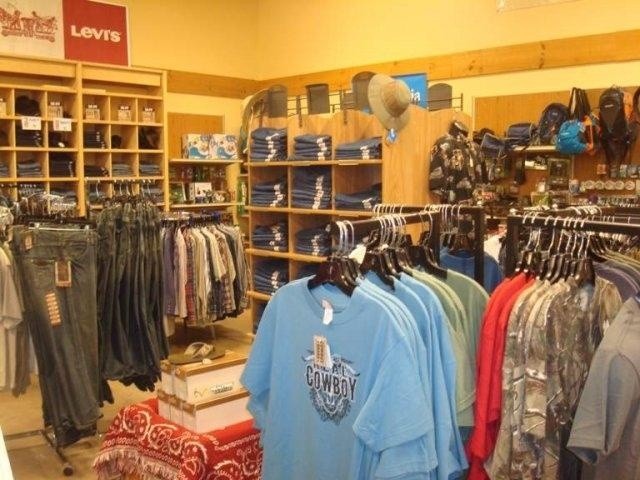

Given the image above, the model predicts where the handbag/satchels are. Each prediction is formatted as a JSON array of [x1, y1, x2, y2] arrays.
[[473, 89, 636, 161]]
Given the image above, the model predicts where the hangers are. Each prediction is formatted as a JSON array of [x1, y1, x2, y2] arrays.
[[0, 177, 162, 230], [359, 203, 474, 296], [308, 220, 364, 297], [490, 195, 637, 297]]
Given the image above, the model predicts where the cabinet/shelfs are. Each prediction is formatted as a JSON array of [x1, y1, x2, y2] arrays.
[[244, 103, 430, 346], [169, 157, 244, 211], [0, 54, 168, 219]]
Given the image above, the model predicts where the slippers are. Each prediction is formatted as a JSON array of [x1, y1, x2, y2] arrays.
[[168, 341, 224, 366]]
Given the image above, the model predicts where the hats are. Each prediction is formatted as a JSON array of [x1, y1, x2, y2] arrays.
[[367, 73, 410, 132]]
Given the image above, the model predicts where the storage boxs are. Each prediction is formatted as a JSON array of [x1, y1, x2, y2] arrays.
[[156, 348, 252, 432]]
